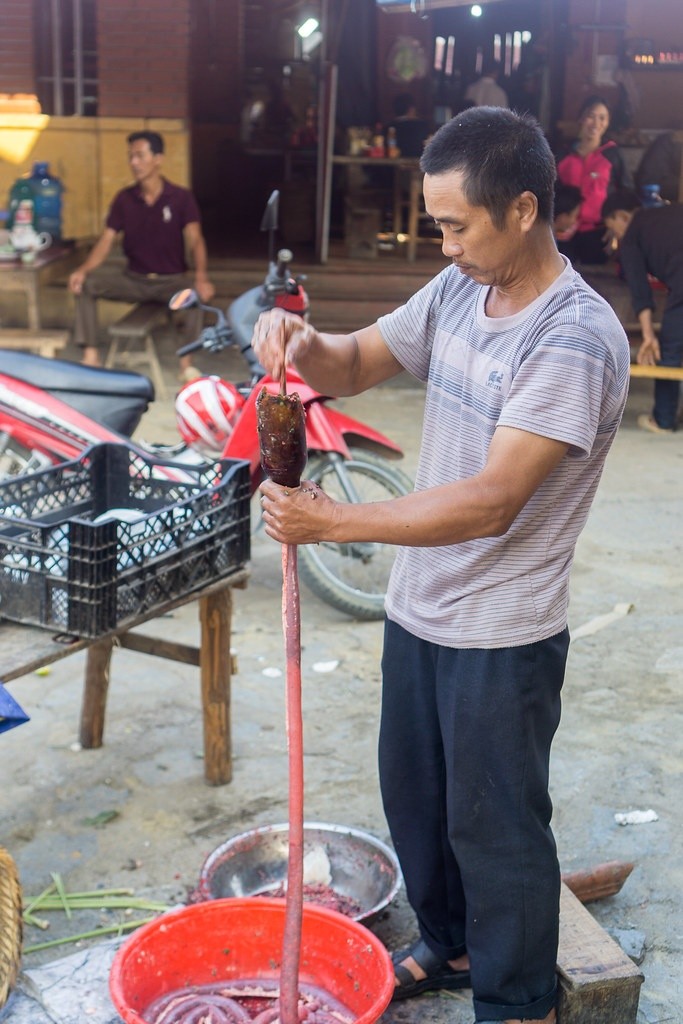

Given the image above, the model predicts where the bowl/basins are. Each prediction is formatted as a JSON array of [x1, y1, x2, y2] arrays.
[[113, 821, 403, 1022], [109, 908, 111, 914]]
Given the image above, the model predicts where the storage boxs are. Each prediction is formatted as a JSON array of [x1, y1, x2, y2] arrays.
[[0, 440, 252, 642]]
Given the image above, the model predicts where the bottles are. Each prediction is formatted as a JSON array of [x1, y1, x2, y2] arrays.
[[10, 161, 63, 248], [258, 390, 307, 491], [372, 120, 398, 158]]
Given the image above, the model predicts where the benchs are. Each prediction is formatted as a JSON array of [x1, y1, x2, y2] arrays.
[[2, 329, 69, 360], [341, 192, 381, 266], [101, 300, 171, 399], [629, 364, 683, 382]]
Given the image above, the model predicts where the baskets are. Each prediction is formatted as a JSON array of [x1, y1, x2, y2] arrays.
[[0, 442, 252, 642]]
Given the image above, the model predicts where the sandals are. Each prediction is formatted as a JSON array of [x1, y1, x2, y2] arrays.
[[386, 937, 474, 999]]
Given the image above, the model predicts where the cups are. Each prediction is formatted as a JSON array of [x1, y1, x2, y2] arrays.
[[642, 184, 660, 210]]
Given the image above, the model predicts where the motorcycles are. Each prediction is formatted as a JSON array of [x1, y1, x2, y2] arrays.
[[0, 190, 414, 623]]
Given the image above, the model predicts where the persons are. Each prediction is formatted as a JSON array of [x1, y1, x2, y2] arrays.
[[601, 189, 683, 434], [554, 183, 587, 234], [634, 120, 683, 206], [374, 92, 442, 236], [555, 94, 636, 281], [68, 129, 215, 383], [463, 59, 511, 111], [251, 106, 630, 1024]]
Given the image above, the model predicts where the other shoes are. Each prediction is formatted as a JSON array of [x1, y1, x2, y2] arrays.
[[178, 366, 205, 382], [637, 413, 675, 433]]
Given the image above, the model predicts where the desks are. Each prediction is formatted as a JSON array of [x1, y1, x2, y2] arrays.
[[0, 567, 251, 782], [329, 153, 446, 261], [1, 237, 86, 329]]
